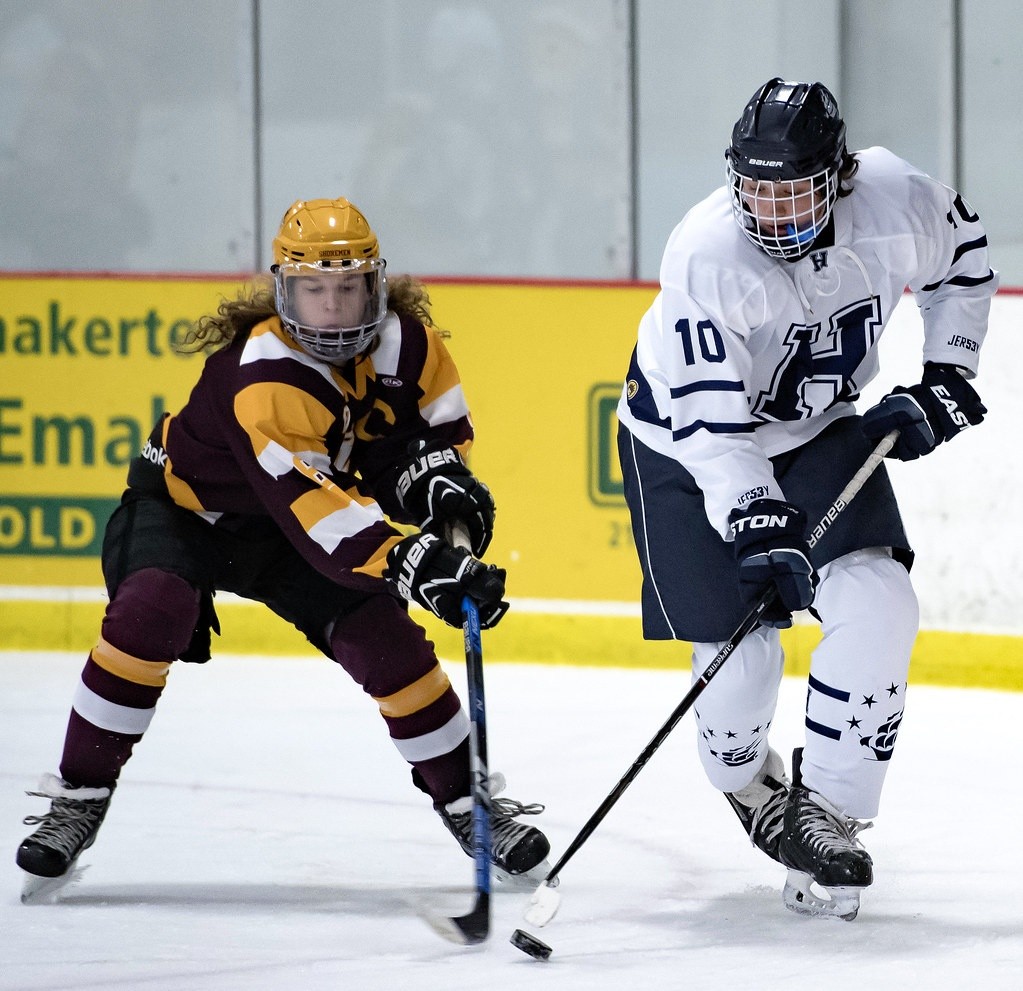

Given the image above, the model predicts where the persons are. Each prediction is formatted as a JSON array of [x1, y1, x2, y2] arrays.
[[14, 196, 561, 903], [617, 81, 1000, 923]]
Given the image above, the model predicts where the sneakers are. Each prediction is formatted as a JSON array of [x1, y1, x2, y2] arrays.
[[777, 748, 874, 922], [433, 789, 555, 889], [723, 750, 791, 864], [15, 773, 111, 908]]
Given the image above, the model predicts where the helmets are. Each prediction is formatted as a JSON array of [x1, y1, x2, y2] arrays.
[[723, 78, 847, 263], [271, 199, 387, 364]]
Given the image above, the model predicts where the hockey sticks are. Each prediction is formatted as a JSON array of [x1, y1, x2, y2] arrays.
[[521, 428, 901, 927], [411, 518, 493, 946]]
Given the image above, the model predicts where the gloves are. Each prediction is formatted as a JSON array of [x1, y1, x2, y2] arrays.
[[861, 361, 988, 463], [395, 444, 498, 558], [382, 537, 509, 630], [728, 498, 819, 630]]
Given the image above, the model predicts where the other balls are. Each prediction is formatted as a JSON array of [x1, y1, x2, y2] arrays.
[[507, 928, 552, 962]]
